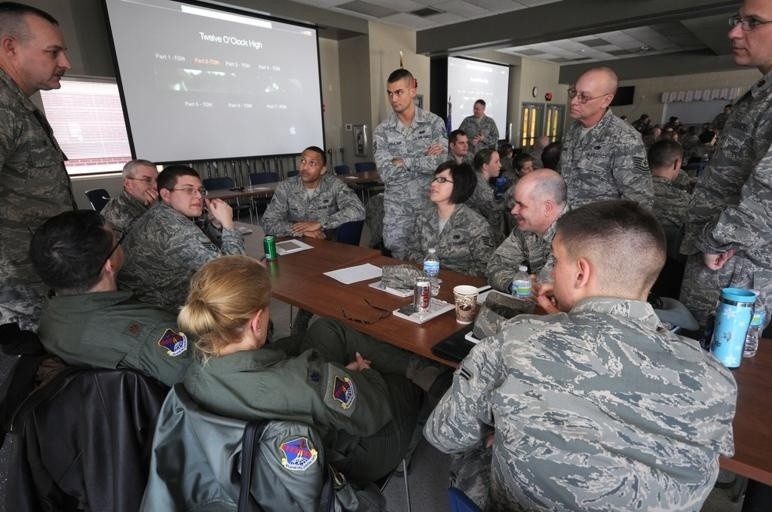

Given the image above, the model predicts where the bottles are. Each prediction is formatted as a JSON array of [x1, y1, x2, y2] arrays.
[[511, 265, 533, 300], [742, 288, 767, 359], [422, 248, 441, 297], [493, 177, 507, 199]]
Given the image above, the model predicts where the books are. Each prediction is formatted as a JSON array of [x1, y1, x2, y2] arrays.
[[368, 275, 442, 298], [392, 297, 455, 324]]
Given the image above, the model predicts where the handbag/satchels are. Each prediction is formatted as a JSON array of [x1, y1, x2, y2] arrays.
[[318, 480, 388, 512]]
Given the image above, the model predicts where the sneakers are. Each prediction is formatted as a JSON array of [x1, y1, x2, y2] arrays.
[[393, 452, 413, 477], [714, 469, 737, 488], [406, 359, 456, 397]]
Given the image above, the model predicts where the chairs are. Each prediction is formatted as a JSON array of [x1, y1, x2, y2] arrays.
[[288, 170, 299, 177], [203, 177, 252, 224], [250, 172, 279, 225], [660, 216, 687, 301], [139, 382, 411, 512], [84, 189, 111, 211], [0, 322, 173, 512], [447, 488, 482, 512], [355, 162, 385, 197], [326, 220, 363, 246], [334, 165, 365, 206]]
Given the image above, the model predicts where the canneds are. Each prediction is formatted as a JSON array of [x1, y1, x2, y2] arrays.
[[413, 276, 430, 313], [264, 236, 276, 261]]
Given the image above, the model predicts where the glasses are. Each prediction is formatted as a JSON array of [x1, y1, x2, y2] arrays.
[[98, 221, 130, 277], [567, 87, 609, 104], [648, 294, 663, 308], [127, 177, 156, 185], [728, 15, 772, 32], [430, 177, 455, 183], [335, 296, 392, 325], [168, 184, 209, 199]]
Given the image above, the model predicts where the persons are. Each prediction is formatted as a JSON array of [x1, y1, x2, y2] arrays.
[[100, 160, 156, 248], [488, 167, 572, 312], [648, 136, 688, 227], [30, 209, 299, 388], [531, 136, 549, 170], [620, 115, 699, 146], [502, 140, 561, 232], [464, 148, 502, 230], [176, 256, 440, 488], [713, 103, 732, 131], [687, 125, 717, 180], [375, 68, 448, 259], [678, 0, 770, 490], [1, 1, 77, 337], [117, 165, 245, 313], [500, 143, 514, 172], [405, 161, 493, 280], [424, 200, 739, 512], [447, 131, 473, 169], [459, 100, 499, 155], [559, 68, 655, 217], [512, 154, 534, 178], [263, 146, 365, 241]]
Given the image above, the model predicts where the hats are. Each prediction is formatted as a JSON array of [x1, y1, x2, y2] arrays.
[[378, 264, 434, 289], [472, 291, 537, 340], [651, 297, 700, 334]]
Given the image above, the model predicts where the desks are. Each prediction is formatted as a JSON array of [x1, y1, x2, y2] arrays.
[[231, 221, 381, 298], [206, 170, 379, 224], [271, 257, 772, 511]]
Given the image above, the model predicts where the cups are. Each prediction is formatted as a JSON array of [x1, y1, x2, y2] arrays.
[[706, 287, 757, 370], [453, 284, 479, 325]]
